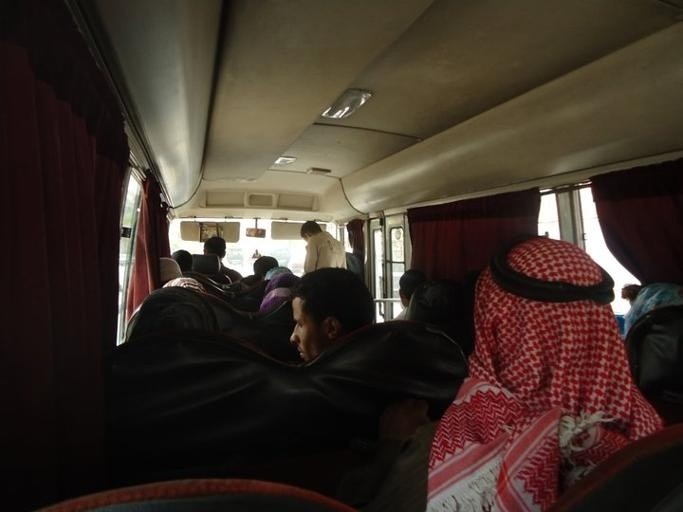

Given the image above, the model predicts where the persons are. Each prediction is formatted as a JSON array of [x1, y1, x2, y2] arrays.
[[159, 276, 208, 296], [252, 254, 278, 280], [287, 264, 376, 364], [330, 234, 671, 512], [170, 248, 194, 275], [202, 236, 244, 287], [299, 220, 346, 273], [614, 275, 682, 429], [390, 266, 426, 327], [257, 264, 301, 314]]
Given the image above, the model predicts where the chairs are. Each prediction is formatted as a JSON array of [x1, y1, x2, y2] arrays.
[[547, 306, 682, 511], [191, 255, 232, 284]]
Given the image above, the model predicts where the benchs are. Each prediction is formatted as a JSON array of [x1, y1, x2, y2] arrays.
[[104, 272, 470, 510]]
